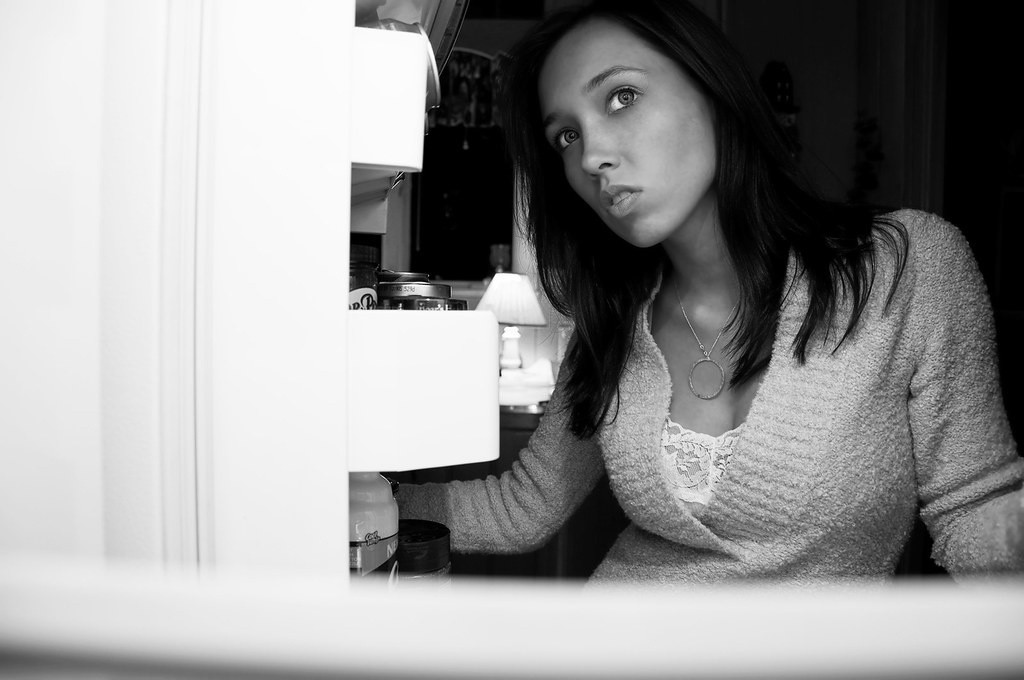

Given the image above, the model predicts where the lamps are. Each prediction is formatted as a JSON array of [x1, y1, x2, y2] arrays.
[[475, 272, 547, 411]]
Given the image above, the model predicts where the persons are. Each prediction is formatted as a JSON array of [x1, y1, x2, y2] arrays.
[[388, 0, 1024, 583]]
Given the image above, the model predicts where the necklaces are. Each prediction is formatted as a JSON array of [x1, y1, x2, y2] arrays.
[[674, 280, 740, 398]]
